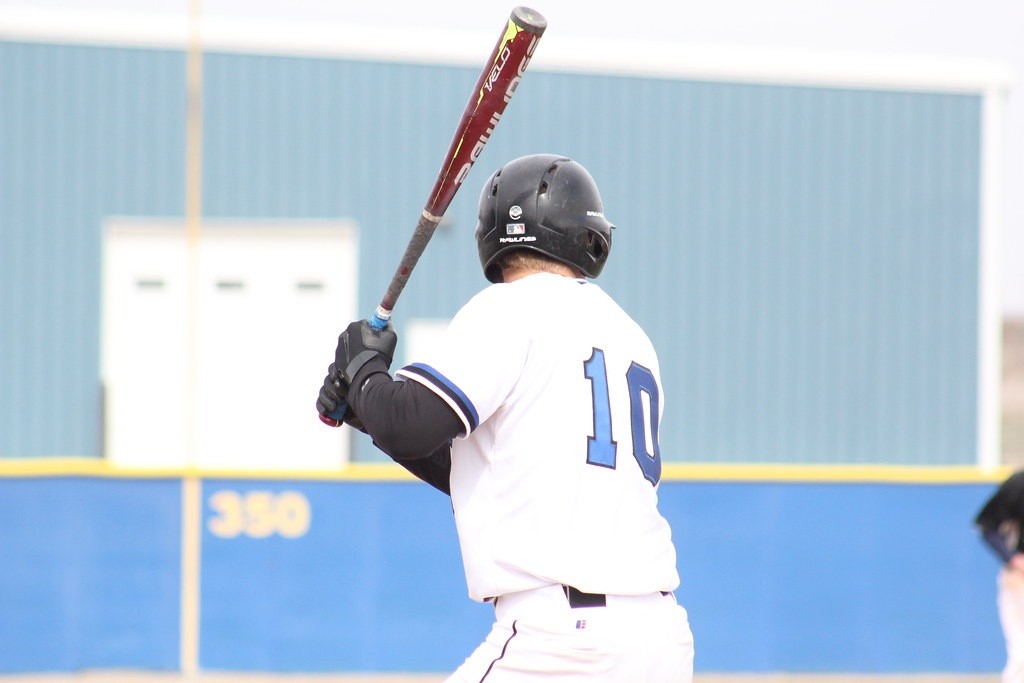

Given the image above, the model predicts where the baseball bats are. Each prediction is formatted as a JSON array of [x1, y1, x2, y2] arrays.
[[318, 5, 549, 428]]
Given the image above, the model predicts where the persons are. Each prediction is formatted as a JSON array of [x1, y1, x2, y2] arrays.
[[317, 153, 694, 683], [976, 471, 1024, 683]]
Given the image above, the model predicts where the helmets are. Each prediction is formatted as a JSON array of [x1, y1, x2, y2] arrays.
[[474, 153, 616, 284]]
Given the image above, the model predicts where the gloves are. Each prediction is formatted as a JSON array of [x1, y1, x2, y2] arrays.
[[334, 319, 398, 386], [315, 362, 364, 431]]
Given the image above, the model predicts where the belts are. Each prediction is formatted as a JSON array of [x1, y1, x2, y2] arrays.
[[563, 586, 669, 609]]
